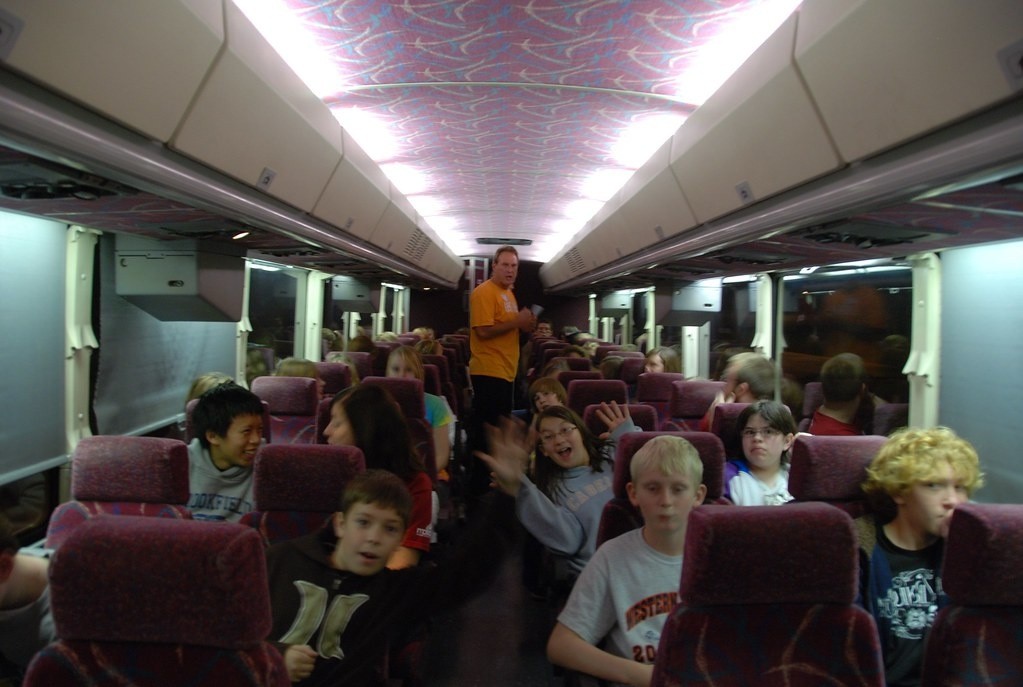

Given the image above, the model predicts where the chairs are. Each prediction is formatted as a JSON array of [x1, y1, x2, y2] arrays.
[[25, 326, 1023, 687]]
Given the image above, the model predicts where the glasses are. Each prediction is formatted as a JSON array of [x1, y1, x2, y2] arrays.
[[741, 428, 781, 437], [540, 425, 577, 442]]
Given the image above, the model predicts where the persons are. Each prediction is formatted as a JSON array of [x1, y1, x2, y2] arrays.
[[468, 246, 537, 469], [251, 326, 367, 352], [853, 427, 985, 687], [347, 327, 470, 355], [521, 319, 599, 373], [0, 346, 798, 687], [807, 336, 908, 436], [634, 332, 754, 381]]
